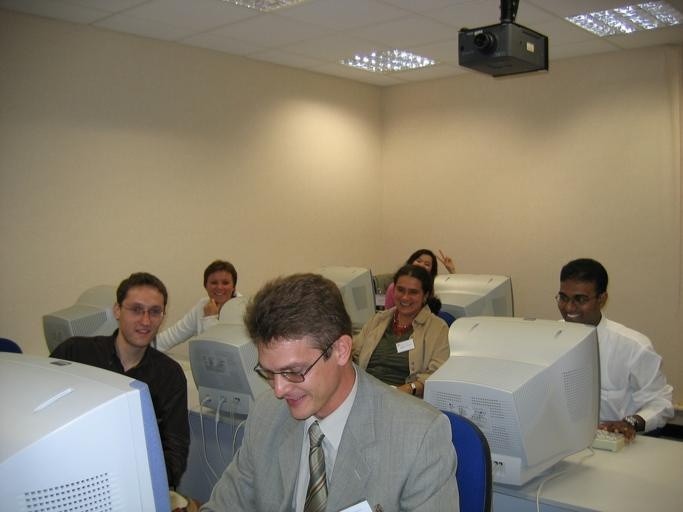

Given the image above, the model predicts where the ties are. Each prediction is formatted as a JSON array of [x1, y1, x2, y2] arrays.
[[303, 420, 329, 511]]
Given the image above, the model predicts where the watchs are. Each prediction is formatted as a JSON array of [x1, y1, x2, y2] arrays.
[[622, 415, 639, 429], [408, 382, 418, 395]]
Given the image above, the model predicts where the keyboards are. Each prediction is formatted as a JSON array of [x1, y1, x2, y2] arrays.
[[586, 428, 626, 453]]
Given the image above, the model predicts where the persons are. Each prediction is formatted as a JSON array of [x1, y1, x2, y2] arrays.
[[196, 272, 463, 512], [545, 256, 676, 444], [148, 260, 266, 352], [381, 247, 458, 326], [37, 272, 191, 490], [337, 263, 452, 400]]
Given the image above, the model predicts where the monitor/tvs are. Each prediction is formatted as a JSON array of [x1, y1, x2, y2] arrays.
[[186, 297, 276, 419], [0, 348, 172, 512], [42, 283, 126, 356], [424, 271, 515, 325], [309, 264, 377, 333], [422, 315, 601, 486]]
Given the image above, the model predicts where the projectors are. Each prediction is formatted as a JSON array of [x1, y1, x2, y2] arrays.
[[458, 22, 549, 77]]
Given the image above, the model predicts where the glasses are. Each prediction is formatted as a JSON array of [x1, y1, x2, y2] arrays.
[[119, 304, 166, 318], [555, 292, 601, 307], [253, 336, 341, 383]]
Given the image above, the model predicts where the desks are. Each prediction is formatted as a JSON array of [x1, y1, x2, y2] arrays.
[[162, 352, 681, 511]]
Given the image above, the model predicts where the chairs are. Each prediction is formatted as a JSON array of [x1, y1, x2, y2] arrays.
[[438, 409, 492, 511]]
